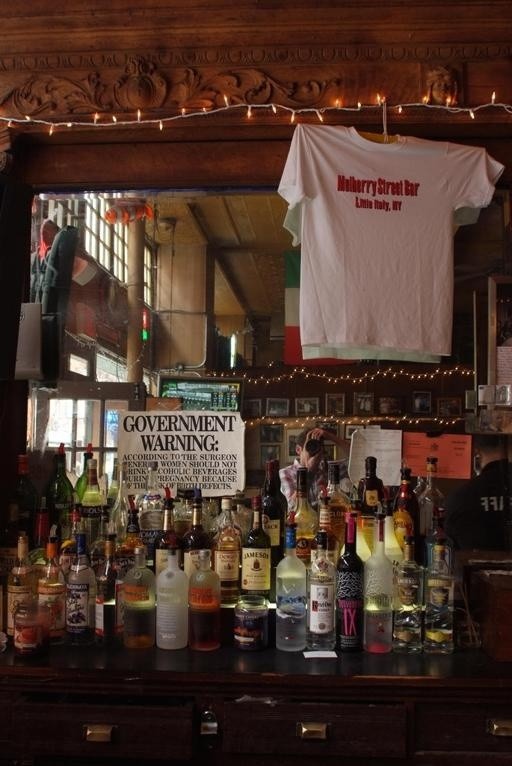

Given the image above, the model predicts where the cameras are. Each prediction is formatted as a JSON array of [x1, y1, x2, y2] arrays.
[[304, 438, 322, 454]]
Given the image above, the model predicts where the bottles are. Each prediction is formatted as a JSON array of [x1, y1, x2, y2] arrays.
[[11, 598, 51, 657], [412, 473, 427, 496], [393, 534, 422, 653], [37, 441, 120, 540], [8, 442, 242, 586], [423, 536, 457, 654], [271, 459, 288, 513], [240, 454, 449, 592], [233, 593, 269, 653], [275, 512, 392, 655], [7, 530, 222, 652]]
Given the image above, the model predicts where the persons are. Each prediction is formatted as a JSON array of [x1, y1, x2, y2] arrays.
[[266, 392, 463, 418], [440, 432, 512, 553], [277, 425, 351, 514]]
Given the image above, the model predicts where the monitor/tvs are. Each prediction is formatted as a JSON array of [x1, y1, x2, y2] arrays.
[[156, 375, 245, 413]]
